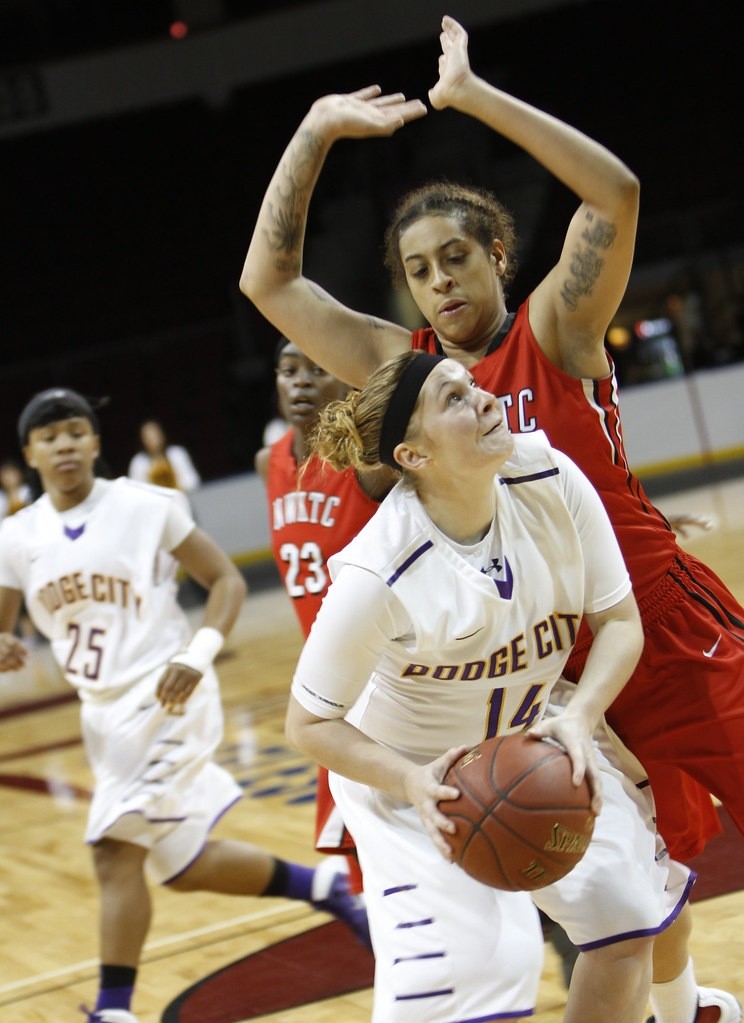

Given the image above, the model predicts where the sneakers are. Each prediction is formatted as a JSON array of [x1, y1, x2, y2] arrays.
[[644, 986, 743, 1023], [309, 855, 371, 949], [78, 1003, 139, 1023]]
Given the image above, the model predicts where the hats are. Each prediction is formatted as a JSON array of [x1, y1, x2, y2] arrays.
[[17, 388, 97, 448]]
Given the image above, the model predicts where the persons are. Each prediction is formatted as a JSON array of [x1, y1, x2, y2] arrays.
[[1, 468, 45, 650], [238, 15, 744, 1022], [282, 352, 695, 1023], [257, 338, 399, 899], [128, 422, 207, 602], [0, 388, 374, 1023]]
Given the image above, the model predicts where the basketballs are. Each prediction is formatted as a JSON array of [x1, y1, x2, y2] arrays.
[[435, 733, 596, 893]]
